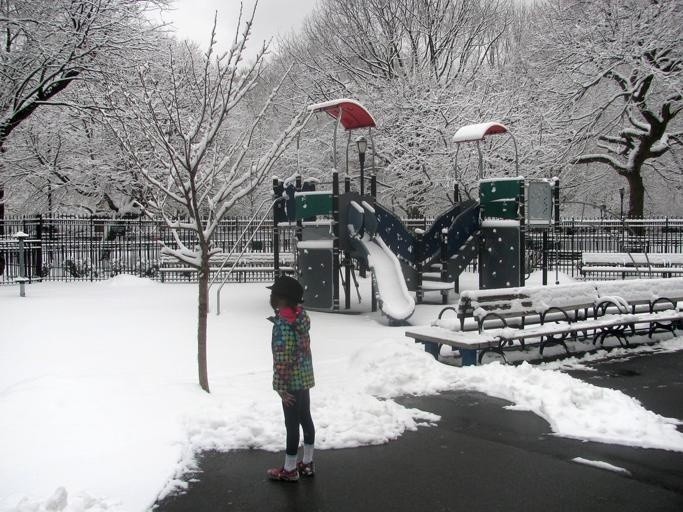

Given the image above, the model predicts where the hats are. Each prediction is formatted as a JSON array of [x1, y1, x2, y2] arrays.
[[266, 275, 305, 304]]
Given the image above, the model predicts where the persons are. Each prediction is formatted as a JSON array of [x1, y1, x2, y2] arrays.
[[266, 276, 314, 482]]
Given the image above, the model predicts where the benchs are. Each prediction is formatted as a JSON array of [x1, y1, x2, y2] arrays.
[[403, 275, 682, 367]]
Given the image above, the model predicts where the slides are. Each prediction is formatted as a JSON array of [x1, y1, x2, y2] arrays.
[[356, 232, 416, 326]]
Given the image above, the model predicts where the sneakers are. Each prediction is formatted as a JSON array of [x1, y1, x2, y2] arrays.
[[266, 464, 300, 481], [297, 460, 315, 476]]
